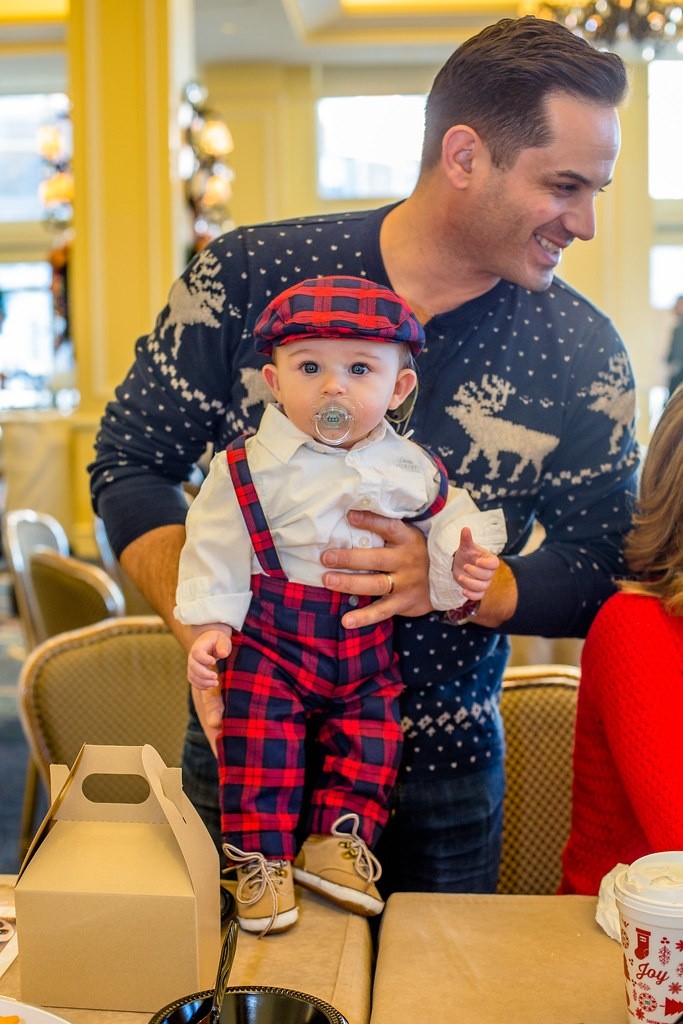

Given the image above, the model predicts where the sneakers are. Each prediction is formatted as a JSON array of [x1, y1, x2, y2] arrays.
[[220, 844, 301, 941], [292, 813, 383, 918]]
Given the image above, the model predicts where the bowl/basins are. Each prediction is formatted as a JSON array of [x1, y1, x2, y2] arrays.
[[148, 986, 349, 1024]]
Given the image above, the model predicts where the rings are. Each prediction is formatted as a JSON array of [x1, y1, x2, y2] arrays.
[[385, 572, 396, 595]]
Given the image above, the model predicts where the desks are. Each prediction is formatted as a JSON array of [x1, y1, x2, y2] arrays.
[[371, 891, 629, 1024], [0, 880, 371, 1024]]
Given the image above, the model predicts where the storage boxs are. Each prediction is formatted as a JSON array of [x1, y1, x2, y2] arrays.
[[14, 740, 221, 1014]]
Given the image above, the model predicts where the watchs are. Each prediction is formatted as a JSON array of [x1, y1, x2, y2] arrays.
[[434, 597, 481, 626]]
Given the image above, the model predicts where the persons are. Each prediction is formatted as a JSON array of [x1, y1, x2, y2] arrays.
[[86, 12, 683, 942]]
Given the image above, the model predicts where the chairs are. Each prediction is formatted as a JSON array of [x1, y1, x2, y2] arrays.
[[0, 509, 71, 658], [18, 615, 189, 804], [18, 543, 127, 860], [500, 664, 581, 895], [95, 464, 205, 615]]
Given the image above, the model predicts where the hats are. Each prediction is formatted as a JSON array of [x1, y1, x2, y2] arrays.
[[254, 276, 426, 357]]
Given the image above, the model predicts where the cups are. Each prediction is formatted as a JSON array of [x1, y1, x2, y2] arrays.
[[613, 850, 683, 1024]]
